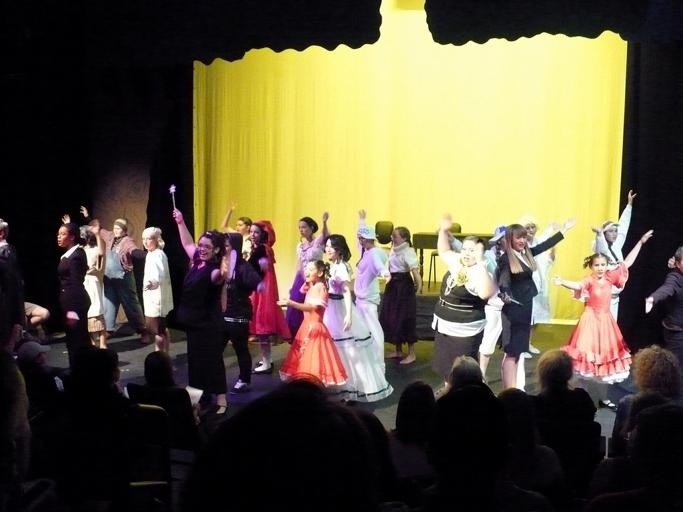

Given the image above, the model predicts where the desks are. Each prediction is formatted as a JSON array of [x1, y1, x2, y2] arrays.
[[412, 231, 496, 296]]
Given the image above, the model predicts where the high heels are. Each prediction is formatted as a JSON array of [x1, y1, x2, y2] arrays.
[[206, 405, 232, 420]]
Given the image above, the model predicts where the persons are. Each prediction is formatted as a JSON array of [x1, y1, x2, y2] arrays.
[[285, 211, 330, 346], [496, 219, 576, 387], [0, 342, 201, 512], [220, 202, 257, 342], [323, 235, 393, 404], [591, 189, 637, 324], [81, 207, 153, 346], [448, 356, 482, 389], [57, 224, 90, 370], [62, 217, 105, 348], [607, 344, 681, 460], [276, 258, 348, 385], [142, 228, 176, 369], [381, 227, 423, 363], [590, 405, 683, 512], [645, 246, 683, 363], [377, 386, 436, 485], [431, 213, 499, 397], [496, 389, 561, 497], [178, 372, 383, 512], [350, 408, 416, 512], [173, 209, 227, 423], [444, 226, 507, 383], [22, 300, 61, 338], [533, 348, 596, 458], [416, 385, 551, 512], [247, 221, 290, 373], [218, 232, 261, 394], [552, 229, 653, 412], [0, 218, 27, 353], [353, 209, 391, 376], [522, 218, 556, 359]]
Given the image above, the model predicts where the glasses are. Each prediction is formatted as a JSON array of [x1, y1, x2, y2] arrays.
[[56, 231, 71, 237], [198, 244, 215, 250]]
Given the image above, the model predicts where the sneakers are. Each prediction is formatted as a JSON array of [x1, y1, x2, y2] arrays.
[[400, 356, 416, 363], [528, 344, 540, 354], [96, 332, 111, 341], [523, 352, 532, 359], [387, 353, 402, 357], [230, 379, 249, 394], [141, 334, 150, 343]]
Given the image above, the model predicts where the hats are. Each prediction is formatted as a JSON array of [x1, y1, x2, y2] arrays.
[[489, 225, 507, 244], [18, 341, 50, 361], [601, 220, 620, 233], [357, 225, 376, 240], [115, 217, 128, 231]]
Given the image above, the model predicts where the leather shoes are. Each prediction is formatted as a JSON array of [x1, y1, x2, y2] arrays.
[[250, 367, 272, 376], [257, 361, 274, 367], [598, 399, 618, 412]]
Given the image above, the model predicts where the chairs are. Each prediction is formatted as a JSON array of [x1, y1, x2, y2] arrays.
[[428, 222, 461, 291], [374, 220, 393, 251], [127, 382, 193, 419]]
